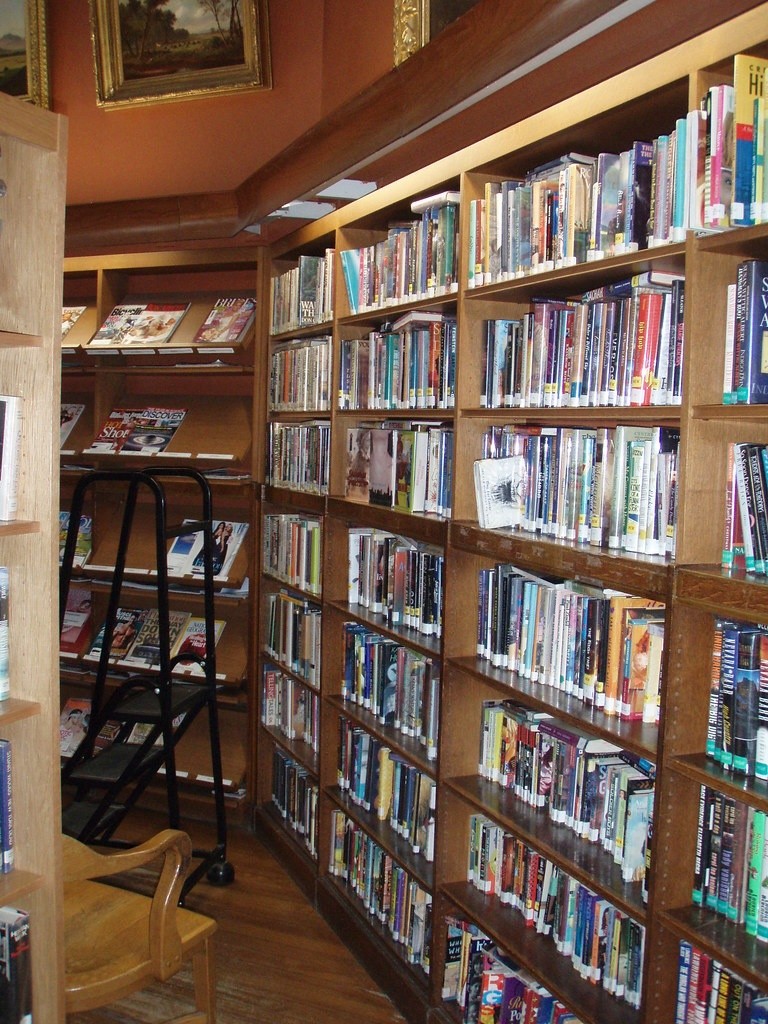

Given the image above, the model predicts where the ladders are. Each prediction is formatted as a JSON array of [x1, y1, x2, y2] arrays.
[[60, 466, 235, 907]]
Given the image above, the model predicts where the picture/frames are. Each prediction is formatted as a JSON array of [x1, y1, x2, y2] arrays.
[[87, 0, 273, 112], [0, 0, 50, 110]]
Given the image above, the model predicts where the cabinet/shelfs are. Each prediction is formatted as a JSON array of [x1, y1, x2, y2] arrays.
[[244, 2, 768, 1024], [61, 245, 260, 830], [0, 91, 68, 1023]]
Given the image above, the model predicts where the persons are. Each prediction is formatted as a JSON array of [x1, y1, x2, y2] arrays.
[[192, 521, 226, 574], [199, 298, 245, 342], [217, 524, 234, 562], [112, 612, 140, 650], [71, 713, 90, 750], [60, 709, 83, 751], [61, 312, 72, 332]]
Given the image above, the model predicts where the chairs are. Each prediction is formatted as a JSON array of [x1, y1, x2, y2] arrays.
[[62, 826, 219, 1023]]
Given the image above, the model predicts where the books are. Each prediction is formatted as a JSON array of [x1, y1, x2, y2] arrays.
[[0, 396, 33, 1024], [60, 297, 257, 759], [260, 54, 768, 1024]]
